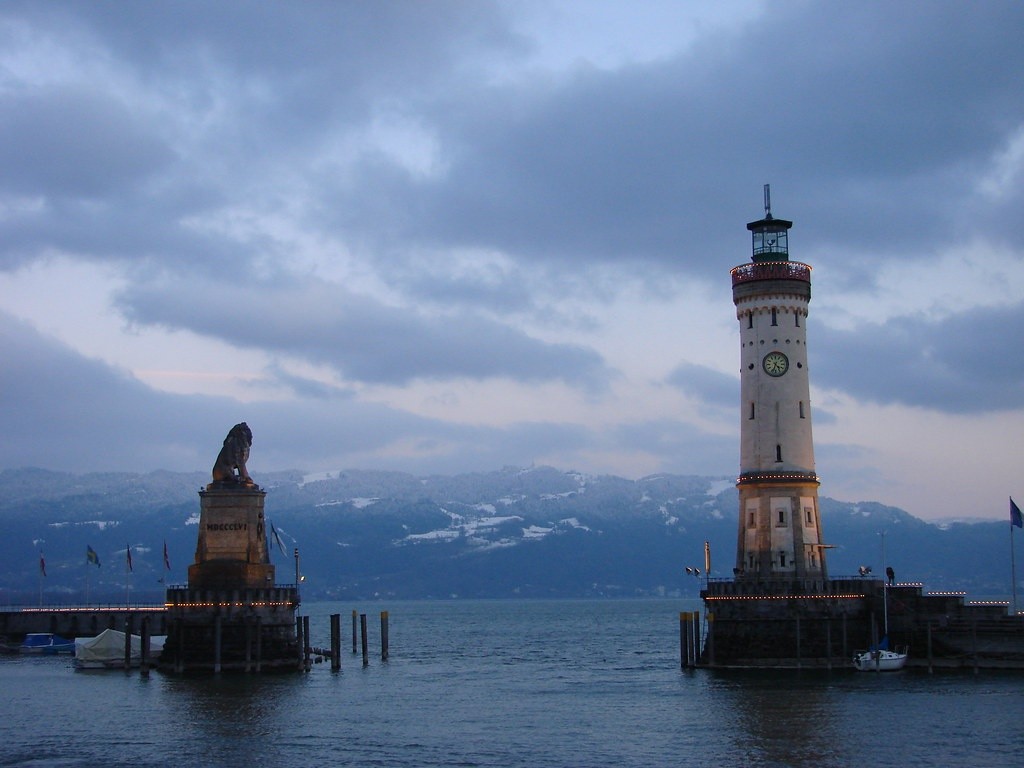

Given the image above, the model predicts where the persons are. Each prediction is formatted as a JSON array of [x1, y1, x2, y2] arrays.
[[886, 567, 895, 587]]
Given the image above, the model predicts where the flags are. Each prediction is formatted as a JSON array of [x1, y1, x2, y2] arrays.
[[165, 544, 173, 571], [41, 549, 47, 576], [272, 525, 287, 558], [126, 545, 133, 571], [1010, 500, 1024, 528], [87, 545, 101, 568]]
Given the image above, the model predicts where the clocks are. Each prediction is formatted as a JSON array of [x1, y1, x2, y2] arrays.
[[762, 351, 789, 377]]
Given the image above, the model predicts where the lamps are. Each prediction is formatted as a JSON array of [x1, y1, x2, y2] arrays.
[[858, 566, 872, 575], [686, 567, 700, 577]]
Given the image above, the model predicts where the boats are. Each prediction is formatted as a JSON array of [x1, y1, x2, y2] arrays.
[[73, 629, 165, 669], [18, 632, 75, 654], [852, 650, 909, 671]]
